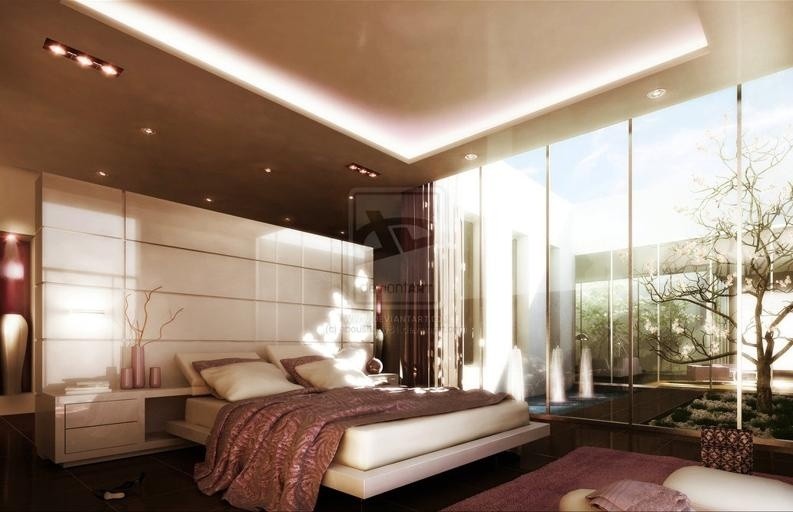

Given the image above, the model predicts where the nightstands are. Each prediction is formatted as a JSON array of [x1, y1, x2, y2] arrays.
[[35, 385, 211, 468], [367, 373, 399, 384]]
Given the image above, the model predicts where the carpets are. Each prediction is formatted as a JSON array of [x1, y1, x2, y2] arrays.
[[438, 445, 793, 512]]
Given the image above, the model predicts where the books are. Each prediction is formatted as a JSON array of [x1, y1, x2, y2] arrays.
[[64, 380, 112, 394]]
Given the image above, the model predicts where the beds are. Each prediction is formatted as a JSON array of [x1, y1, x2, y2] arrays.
[[166, 384, 551, 512]]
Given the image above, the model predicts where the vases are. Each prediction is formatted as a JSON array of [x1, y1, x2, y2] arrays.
[[120, 347, 162, 389]]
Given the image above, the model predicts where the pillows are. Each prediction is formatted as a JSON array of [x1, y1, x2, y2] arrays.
[[192, 356, 379, 403]]
